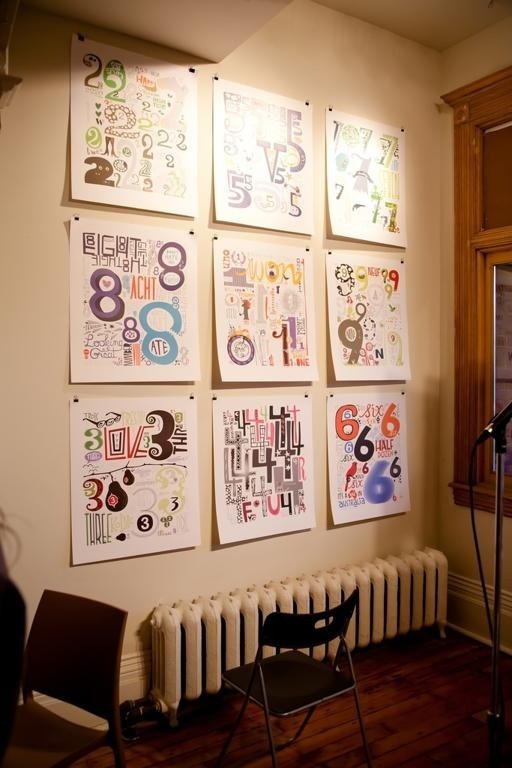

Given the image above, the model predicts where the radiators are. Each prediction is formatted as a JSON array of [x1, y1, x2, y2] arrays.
[[144, 545, 452, 726]]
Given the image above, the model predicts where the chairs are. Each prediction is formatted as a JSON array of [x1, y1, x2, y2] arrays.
[[1, 587, 132, 768], [218, 587, 375, 767]]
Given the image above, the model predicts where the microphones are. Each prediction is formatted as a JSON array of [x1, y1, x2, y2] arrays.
[[476, 399, 512, 443]]
